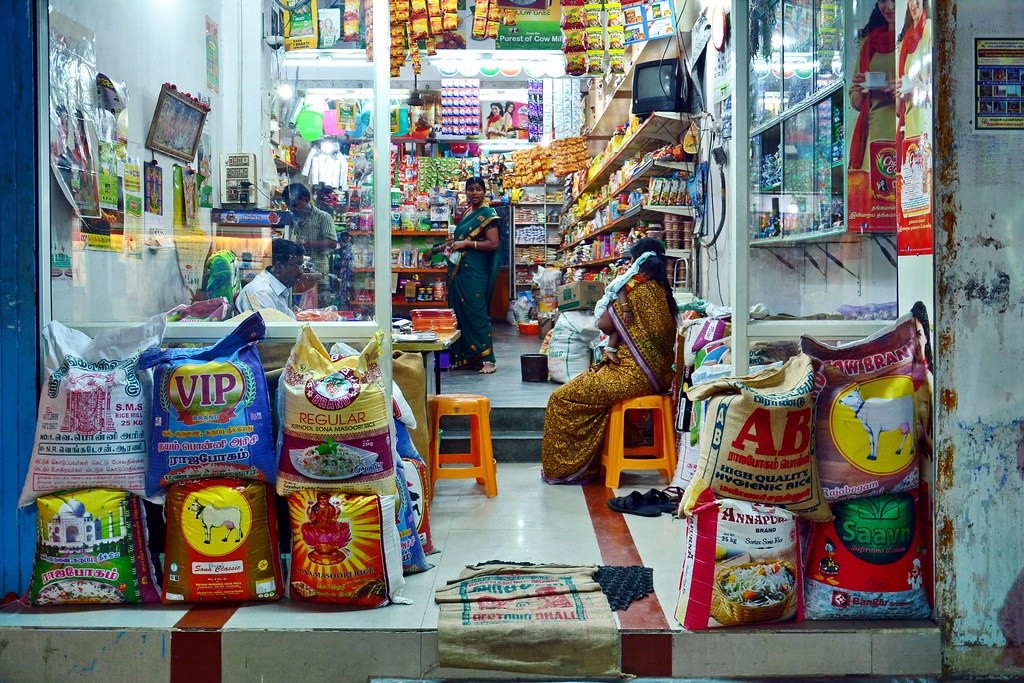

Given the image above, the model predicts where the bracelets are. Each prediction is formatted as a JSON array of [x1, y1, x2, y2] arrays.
[[474, 241, 477, 248], [431, 244, 441, 254], [621, 303, 628, 306]]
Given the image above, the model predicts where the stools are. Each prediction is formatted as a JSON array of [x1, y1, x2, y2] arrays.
[[600, 390, 678, 491], [428, 393, 499, 500]]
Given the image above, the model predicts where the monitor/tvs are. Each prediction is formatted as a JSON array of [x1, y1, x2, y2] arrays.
[[632, 56, 689, 121]]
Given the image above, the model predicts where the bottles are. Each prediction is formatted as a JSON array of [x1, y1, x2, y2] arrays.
[[401, 202, 416, 232], [416, 191, 430, 231], [417, 276, 448, 302]]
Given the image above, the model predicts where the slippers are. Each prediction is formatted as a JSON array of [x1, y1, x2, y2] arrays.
[[643, 488, 677, 513], [608, 490, 661, 517]]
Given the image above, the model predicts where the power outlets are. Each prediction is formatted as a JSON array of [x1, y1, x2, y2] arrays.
[[219, 153, 256, 205]]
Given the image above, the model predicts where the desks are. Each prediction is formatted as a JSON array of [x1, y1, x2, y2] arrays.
[[393, 328, 463, 393]]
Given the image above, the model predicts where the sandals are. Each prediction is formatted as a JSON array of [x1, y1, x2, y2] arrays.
[[663, 486, 685, 502]]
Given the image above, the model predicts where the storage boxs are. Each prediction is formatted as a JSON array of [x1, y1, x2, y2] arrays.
[[556, 280, 605, 313], [537, 316, 552, 340], [517, 323, 540, 335]]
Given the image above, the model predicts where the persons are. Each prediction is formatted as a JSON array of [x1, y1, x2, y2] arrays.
[[280, 182, 339, 308], [604, 255, 664, 363], [317, 19, 339, 44], [540, 237, 679, 486], [848, 0, 934, 174], [910, 300, 932, 520], [235, 238, 305, 320], [51, 104, 99, 216], [423, 176, 502, 374], [486, 102, 515, 139]]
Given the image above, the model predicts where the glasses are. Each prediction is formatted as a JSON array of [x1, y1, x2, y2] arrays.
[[287, 263, 303, 270]]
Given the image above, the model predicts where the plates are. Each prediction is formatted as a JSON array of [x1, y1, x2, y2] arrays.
[[289, 442, 378, 481]]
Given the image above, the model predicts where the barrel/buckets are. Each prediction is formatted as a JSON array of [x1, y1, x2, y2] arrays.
[[520, 354, 548, 382], [296, 109, 363, 141]]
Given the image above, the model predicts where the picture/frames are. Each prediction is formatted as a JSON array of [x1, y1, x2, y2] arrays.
[[143, 83, 208, 164], [198, 92, 211, 111]]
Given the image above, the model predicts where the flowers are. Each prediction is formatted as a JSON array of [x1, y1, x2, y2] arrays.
[[165, 81, 211, 113]]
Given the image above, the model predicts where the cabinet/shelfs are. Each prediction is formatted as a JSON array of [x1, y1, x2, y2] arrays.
[[346, 228, 453, 310], [745, 0, 898, 251], [563, 108, 703, 305], [514, 180, 563, 300]]
